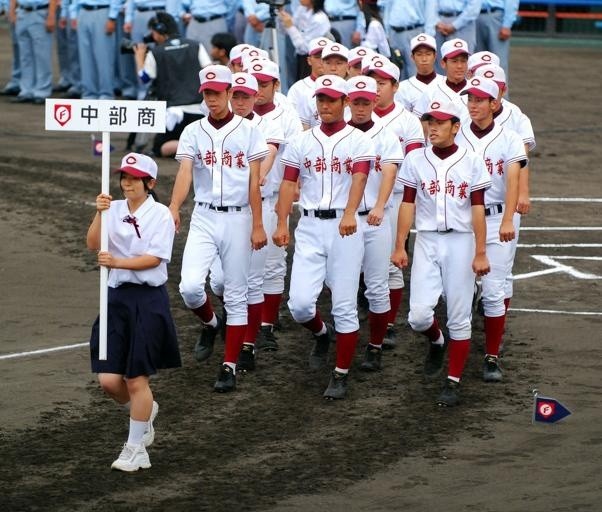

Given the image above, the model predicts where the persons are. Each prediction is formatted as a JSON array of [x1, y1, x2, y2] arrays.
[[87, 152, 181, 474], [391, 27, 536, 407], [168, 43, 304, 392], [1, 0, 521, 106], [132, 11, 237, 161], [272, 37, 424, 399]]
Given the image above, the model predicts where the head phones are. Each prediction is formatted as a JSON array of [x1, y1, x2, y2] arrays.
[[151, 14, 166, 35]]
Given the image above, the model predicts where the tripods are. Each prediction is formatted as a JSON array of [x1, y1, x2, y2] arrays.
[[257, 16, 282, 94]]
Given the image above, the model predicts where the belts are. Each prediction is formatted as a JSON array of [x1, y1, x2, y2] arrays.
[[194, 14, 221, 23], [304, 209, 336, 219], [481, 7, 504, 13], [439, 11, 462, 16], [199, 202, 241, 211], [137, 6, 165, 11], [485, 204, 502, 215], [82, 4, 109, 10], [358, 210, 369, 216], [391, 24, 420, 32], [20, 3, 49, 11], [328, 16, 357, 21]]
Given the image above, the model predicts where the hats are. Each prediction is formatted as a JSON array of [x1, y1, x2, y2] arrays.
[[198, 65, 231, 92], [410, 33, 438, 55], [441, 37, 506, 99], [112, 152, 158, 180], [419, 96, 461, 120], [308, 37, 401, 104], [229, 44, 280, 101]]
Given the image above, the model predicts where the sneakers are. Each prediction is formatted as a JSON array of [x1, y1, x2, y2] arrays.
[[309, 320, 334, 370], [323, 366, 348, 401], [361, 321, 396, 371], [142, 401, 159, 447], [0, 84, 137, 104], [435, 379, 464, 407], [424, 340, 447, 378], [193, 307, 279, 392], [483, 354, 504, 382], [111, 442, 152, 471]]
